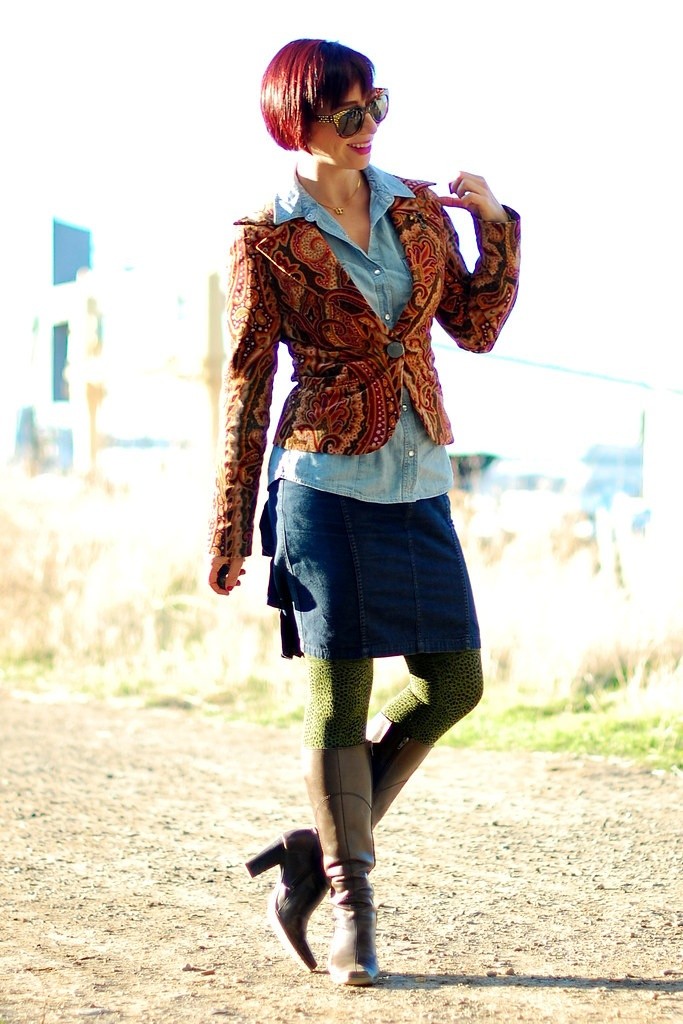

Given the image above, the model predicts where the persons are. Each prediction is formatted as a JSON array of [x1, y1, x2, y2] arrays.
[[207, 39, 521, 986]]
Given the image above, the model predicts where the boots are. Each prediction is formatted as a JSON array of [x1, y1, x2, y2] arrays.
[[244, 710, 434, 987]]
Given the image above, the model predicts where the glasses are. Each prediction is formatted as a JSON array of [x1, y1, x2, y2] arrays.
[[311, 87, 389, 140]]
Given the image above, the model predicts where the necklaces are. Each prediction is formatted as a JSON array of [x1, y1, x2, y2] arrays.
[[318, 176, 362, 215]]
